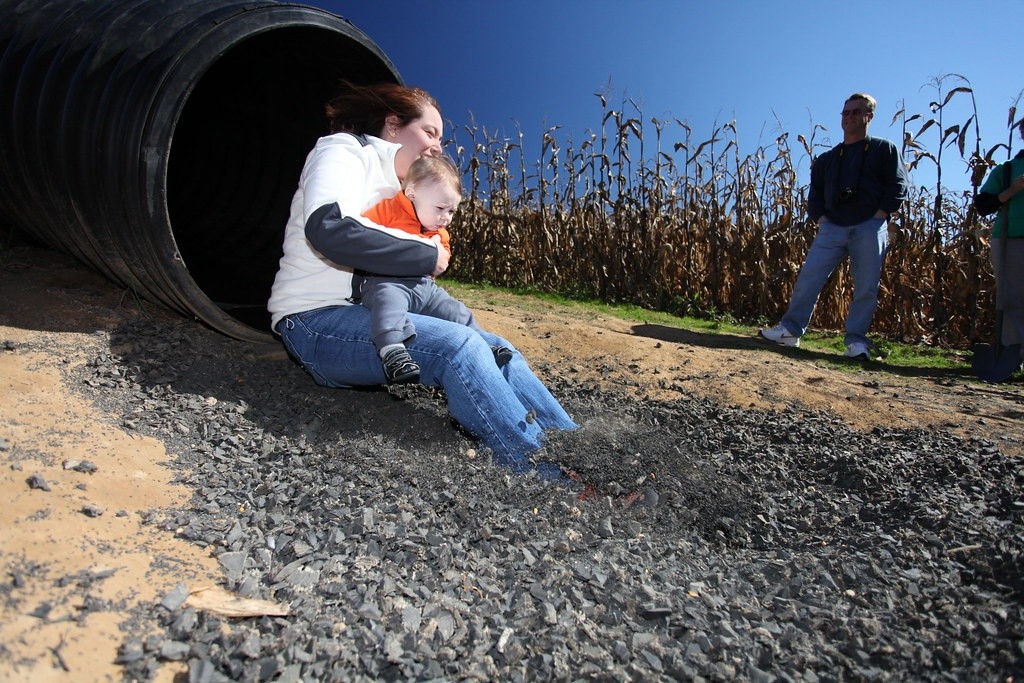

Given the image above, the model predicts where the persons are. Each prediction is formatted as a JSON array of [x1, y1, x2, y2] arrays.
[[756, 91, 907, 358], [264, 86, 660, 511], [357, 150, 514, 387], [974, 116, 1024, 382]]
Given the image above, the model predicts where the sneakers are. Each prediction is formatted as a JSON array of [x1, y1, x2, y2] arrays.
[[758, 321, 800, 347], [381, 346, 420, 381], [490, 345, 513, 370], [843, 341, 871, 360]]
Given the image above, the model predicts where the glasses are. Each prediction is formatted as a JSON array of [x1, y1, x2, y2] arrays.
[[840, 108, 863, 117]]
